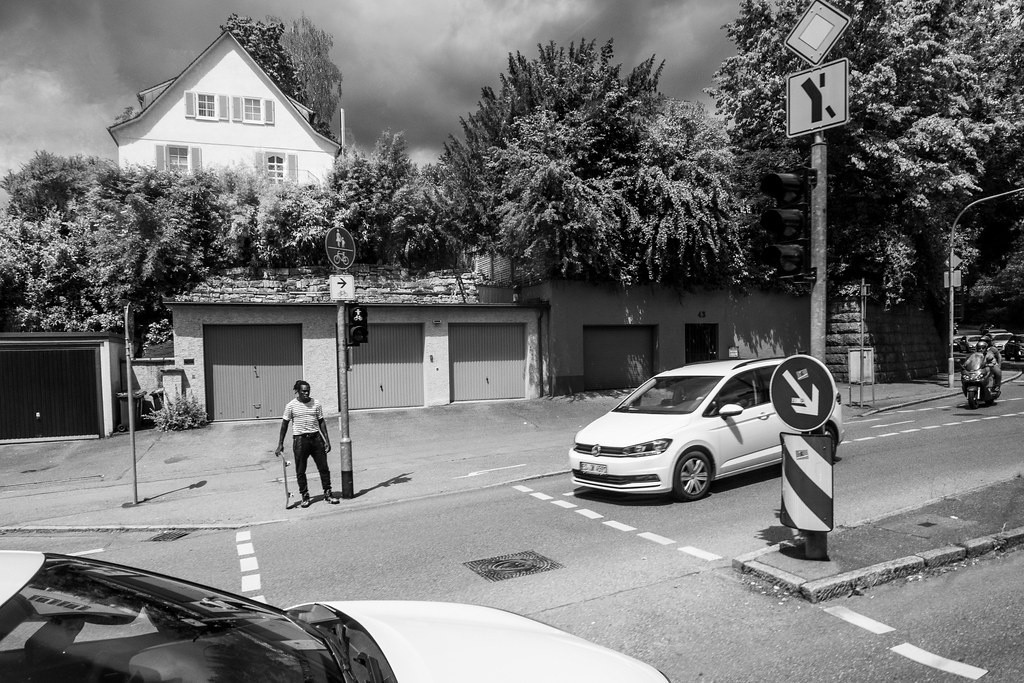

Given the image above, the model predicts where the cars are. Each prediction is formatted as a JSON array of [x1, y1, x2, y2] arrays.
[[953, 323, 1014, 365], [0, 549, 671, 683], [568, 356, 848, 502], [1004, 334, 1024, 361]]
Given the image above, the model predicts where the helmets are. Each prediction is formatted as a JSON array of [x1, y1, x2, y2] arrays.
[[980, 336, 990, 342], [977, 341, 987, 348]]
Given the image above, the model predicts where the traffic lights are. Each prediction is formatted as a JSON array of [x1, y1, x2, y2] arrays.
[[758, 164, 818, 285], [347, 303, 369, 344]]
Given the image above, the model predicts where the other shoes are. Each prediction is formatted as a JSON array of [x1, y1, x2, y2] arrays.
[[324, 490, 339, 504], [301, 493, 309, 507], [992, 386, 1000, 391]]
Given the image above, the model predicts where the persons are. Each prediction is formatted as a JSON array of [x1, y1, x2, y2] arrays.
[[275, 380, 340, 507], [961, 324, 1003, 400]]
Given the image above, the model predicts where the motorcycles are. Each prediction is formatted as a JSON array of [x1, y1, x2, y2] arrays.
[[954, 351, 1001, 409]]
